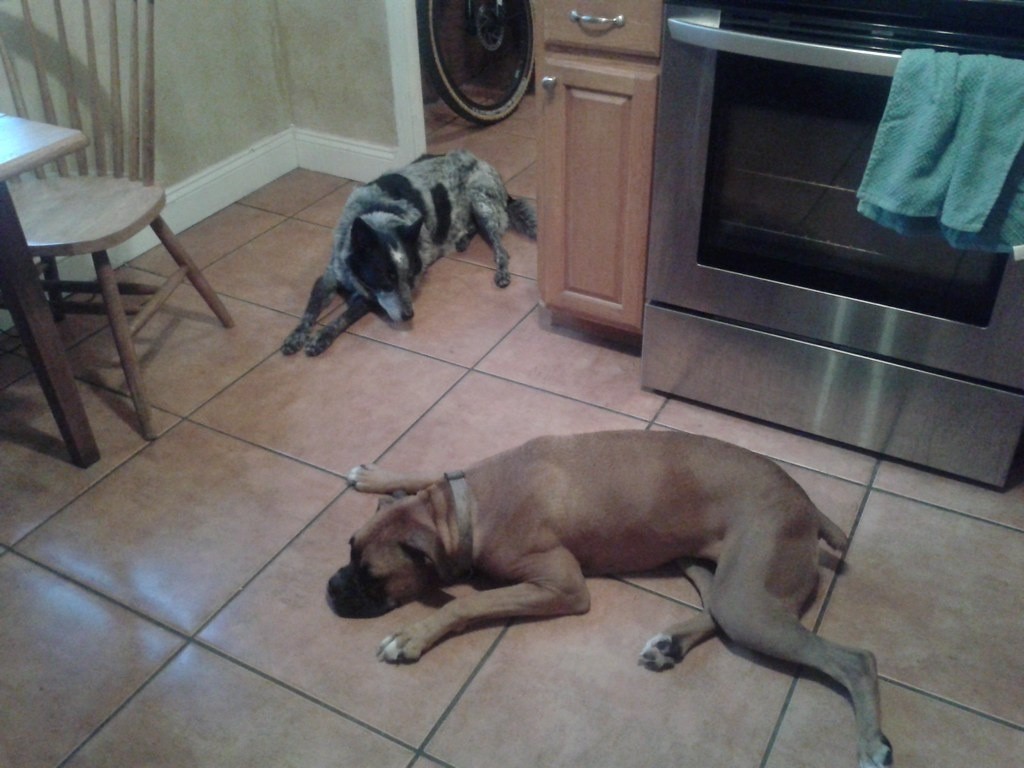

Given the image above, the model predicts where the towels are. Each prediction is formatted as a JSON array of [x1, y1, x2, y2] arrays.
[[856, 41, 1024, 257]]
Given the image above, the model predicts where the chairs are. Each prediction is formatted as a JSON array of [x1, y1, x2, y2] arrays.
[[0, 0, 234, 439]]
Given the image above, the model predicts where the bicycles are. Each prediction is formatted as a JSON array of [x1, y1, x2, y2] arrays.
[[415, 1, 537, 125]]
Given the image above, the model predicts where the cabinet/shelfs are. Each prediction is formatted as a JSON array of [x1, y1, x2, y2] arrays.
[[530, 0, 663, 349]]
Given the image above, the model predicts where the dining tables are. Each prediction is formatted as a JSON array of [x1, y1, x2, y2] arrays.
[[0, 113, 101, 468]]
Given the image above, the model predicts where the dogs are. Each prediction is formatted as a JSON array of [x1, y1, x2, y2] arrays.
[[278, 147, 536, 358], [327, 429, 893, 768]]
